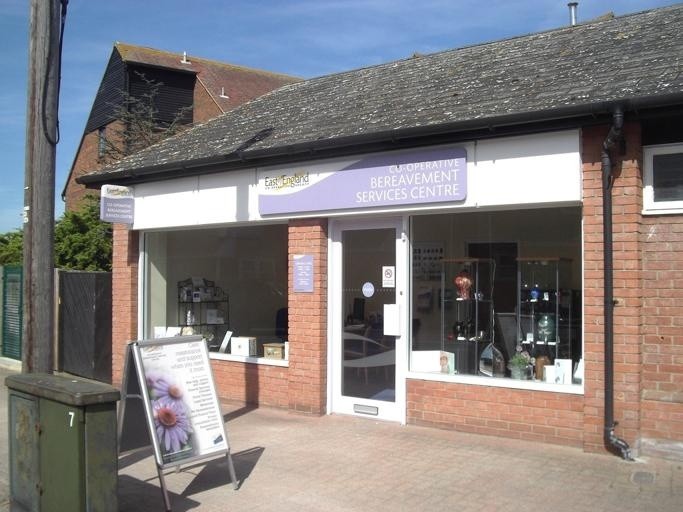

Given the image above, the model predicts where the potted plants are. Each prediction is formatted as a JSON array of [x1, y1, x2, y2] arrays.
[[507, 354, 534, 380]]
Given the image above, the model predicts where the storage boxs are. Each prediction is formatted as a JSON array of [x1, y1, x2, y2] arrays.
[[263, 343, 285, 360], [231, 336, 257, 357]]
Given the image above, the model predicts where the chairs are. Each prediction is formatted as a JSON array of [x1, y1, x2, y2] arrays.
[[369, 336, 395, 381], [345, 327, 374, 384]]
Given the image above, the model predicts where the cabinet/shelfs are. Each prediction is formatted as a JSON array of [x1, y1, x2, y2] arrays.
[[439, 258, 496, 375], [515, 256, 572, 381], [177, 288, 229, 352]]
